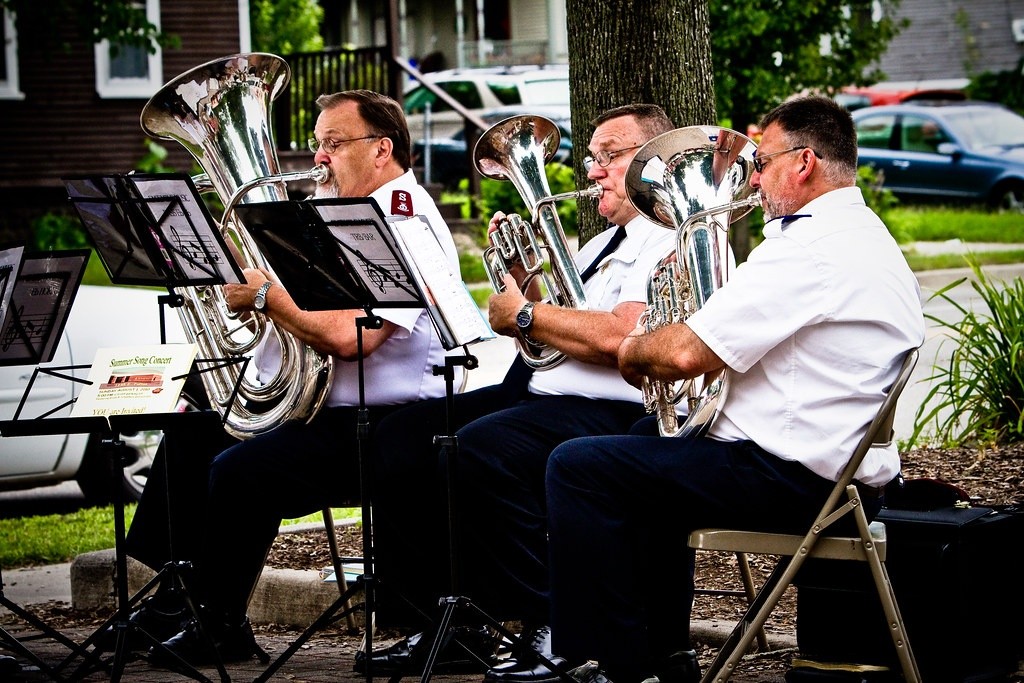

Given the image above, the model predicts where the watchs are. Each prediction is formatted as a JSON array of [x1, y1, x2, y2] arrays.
[[515, 301, 537, 338], [253, 281, 273, 313]]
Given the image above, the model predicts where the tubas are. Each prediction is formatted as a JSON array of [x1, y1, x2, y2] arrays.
[[472, 114, 605, 372], [624, 124, 764, 440], [138, 52, 335, 441]]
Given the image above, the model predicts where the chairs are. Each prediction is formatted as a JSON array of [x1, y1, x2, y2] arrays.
[[685, 348, 923, 683]]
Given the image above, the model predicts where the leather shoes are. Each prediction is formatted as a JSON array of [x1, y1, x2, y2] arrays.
[[483, 625, 568, 683], [92, 597, 192, 651], [146, 616, 257, 668], [354, 631, 490, 676], [579, 651, 702, 682]]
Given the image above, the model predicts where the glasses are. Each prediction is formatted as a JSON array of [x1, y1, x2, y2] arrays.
[[307, 135, 380, 153], [582, 144, 642, 171], [753, 146, 823, 172]]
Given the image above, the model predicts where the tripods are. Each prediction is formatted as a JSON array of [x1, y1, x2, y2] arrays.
[[234, 195, 496, 683], [383, 213, 581, 683], [0, 353, 250, 683]]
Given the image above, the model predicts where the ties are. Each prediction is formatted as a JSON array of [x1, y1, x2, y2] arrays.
[[501, 224, 628, 389]]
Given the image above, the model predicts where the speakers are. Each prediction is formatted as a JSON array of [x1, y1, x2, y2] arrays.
[[795, 511, 1023, 682]]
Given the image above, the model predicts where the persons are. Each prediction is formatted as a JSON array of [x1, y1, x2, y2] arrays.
[[355, 103, 737, 683], [545, 96, 925, 683], [90, 89, 468, 663]]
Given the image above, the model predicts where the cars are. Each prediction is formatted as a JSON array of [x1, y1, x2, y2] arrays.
[[402, 63, 574, 188], [848, 98, 1024, 217], [0, 243, 162, 509]]
